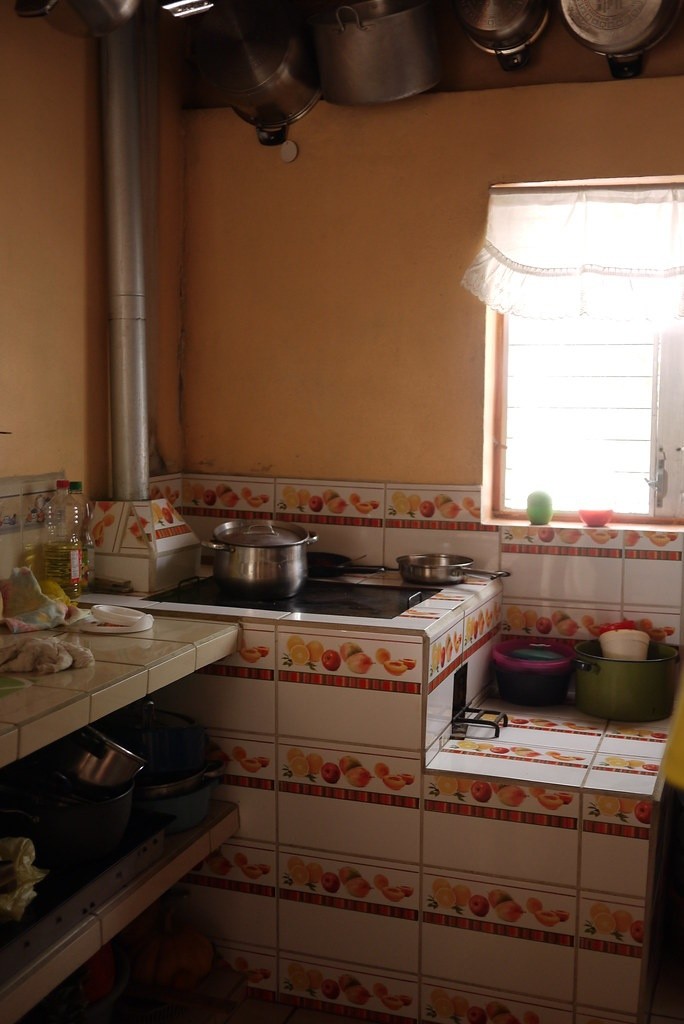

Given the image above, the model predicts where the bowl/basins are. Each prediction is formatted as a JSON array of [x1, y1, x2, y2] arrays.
[[78, 952, 134, 1024], [492, 637, 574, 706], [134, 782, 214, 829]]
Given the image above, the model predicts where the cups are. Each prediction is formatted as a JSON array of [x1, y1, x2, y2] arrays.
[[599, 629, 650, 660]]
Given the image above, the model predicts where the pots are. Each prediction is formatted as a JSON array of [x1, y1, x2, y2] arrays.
[[47, 726, 147, 801], [45, 779, 136, 845], [206, 520, 318, 599], [140, 759, 224, 798], [306, 553, 385, 578], [396, 554, 511, 588], [570, 642, 676, 721]]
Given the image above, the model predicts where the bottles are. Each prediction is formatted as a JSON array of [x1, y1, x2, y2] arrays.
[[62, 482, 94, 587], [43, 480, 81, 599]]
